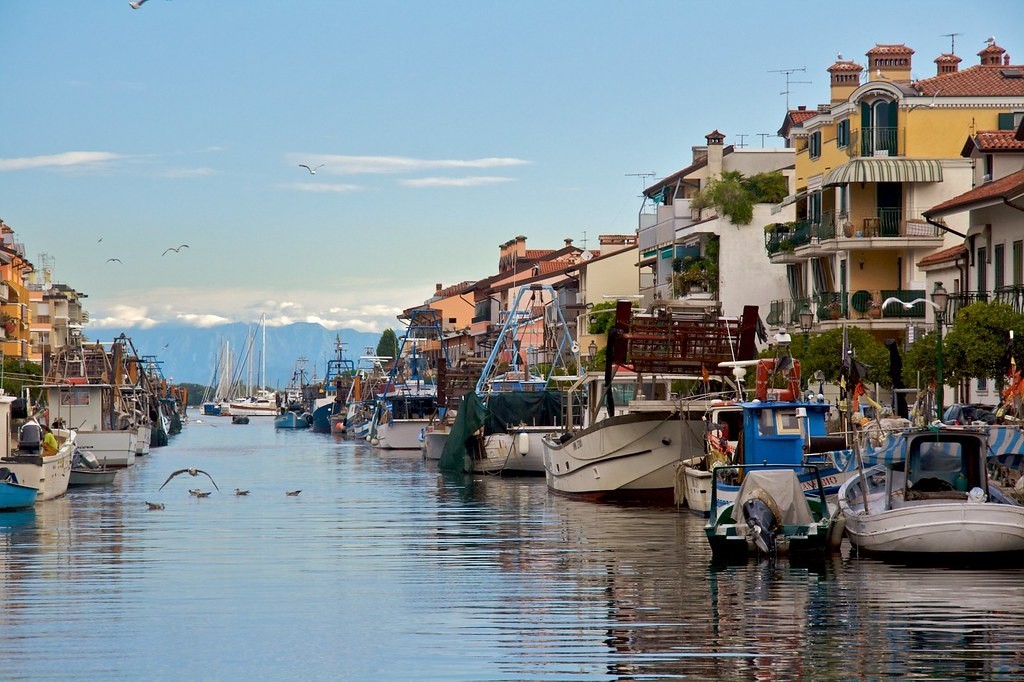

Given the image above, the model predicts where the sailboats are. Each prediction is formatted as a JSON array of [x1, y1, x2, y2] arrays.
[[200, 312, 282, 417]]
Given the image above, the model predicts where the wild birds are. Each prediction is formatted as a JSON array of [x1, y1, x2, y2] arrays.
[[158, 344, 168, 354], [234, 488, 251, 495], [299, 163, 325, 174], [104, 258, 123, 264], [162, 244, 189, 256], [159, 466, 221, 495], [97, 235, 103, 243], [189, 489, 212, 497], [191, 419, 216, 427], [285, 489, 302, 496], [129, 0, 148, 9], [145, 501, 166, 509]]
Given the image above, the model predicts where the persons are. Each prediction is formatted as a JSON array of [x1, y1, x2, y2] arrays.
[[40, 424, 58, 456]]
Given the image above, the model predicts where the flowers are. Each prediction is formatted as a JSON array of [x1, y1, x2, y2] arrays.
[[867, 300, 882, 308], [827, 302, 840, 311]]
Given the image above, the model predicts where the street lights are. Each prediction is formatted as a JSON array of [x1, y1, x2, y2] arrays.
[[588, 341, 597, 372]]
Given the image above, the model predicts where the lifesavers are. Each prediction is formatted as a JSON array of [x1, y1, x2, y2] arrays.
[[560, 432, 576, 442], [66, 377, 86, 384], [755, 358, 801, 402], [612, 364, 634, 371], [712, 402, 732, 406], [45, 408, 49, 424], [306, 415, 313, 422]]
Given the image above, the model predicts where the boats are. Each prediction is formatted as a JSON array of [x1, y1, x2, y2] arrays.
[[0, 328, 188, 502], [703, 460, 840, 565], [542, 372, 739, 501], [270, 308, 479, 461], [672, 322, 882, 518], [457, 284, 587, 479], [838, 430, 1024, 568], [0, 467, 39, 509]]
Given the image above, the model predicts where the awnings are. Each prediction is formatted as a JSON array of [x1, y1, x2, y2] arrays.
[[819, 160, 944, 186], [771, 190, 807, 215]]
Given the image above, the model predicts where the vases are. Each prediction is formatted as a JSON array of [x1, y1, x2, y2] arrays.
[[868, 310, 881, 317], [831, 311, 840, 319]]
